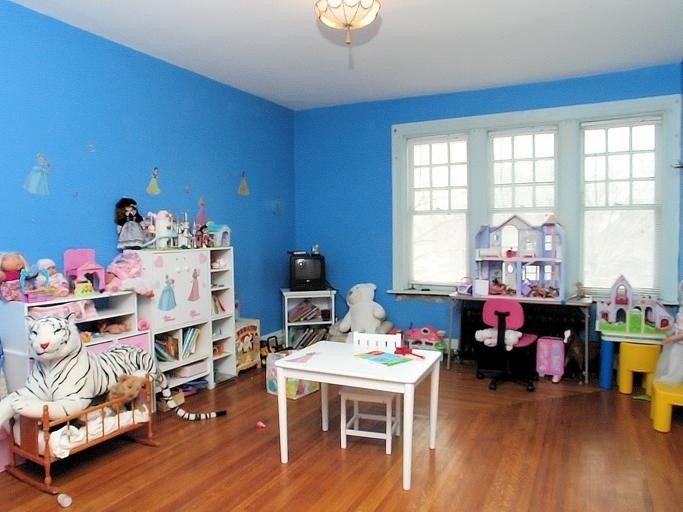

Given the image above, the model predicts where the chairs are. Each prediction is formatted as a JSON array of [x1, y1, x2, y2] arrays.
[[338, 330, 402, 455], [617, 343, 661, 395], [477, 297, 539, 393], [650, 379, 682, 433]]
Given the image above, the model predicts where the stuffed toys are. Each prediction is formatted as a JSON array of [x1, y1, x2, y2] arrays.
[[98, 374, 146, 413], [338, 284, 386, 336], [0, 313, 226, 425], [328, 319, 347, 342], [474, 328, 523, 351], [0, 197, 231, 343]]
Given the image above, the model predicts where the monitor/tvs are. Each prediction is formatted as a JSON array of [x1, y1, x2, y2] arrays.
[[290, 255, 324, 291]]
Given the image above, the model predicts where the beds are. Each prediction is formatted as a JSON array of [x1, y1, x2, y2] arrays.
[[4, 369, 159, 495]]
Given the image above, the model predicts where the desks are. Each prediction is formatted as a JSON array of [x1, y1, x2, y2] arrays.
[[445, 289, 594, 385], [599, 324, 668, 389], [274, 340, 443, 491]]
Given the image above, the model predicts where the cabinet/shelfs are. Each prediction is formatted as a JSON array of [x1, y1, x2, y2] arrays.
[[280, 287, 337, 349], [0, 246, 238, 457]]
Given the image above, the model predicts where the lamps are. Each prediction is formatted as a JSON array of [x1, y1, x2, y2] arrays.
[[313, 0, 382, 45]]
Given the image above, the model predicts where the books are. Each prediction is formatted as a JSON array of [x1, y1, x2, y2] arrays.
[[288, 300, 328, 350], [154, 329, 200, 363]]
